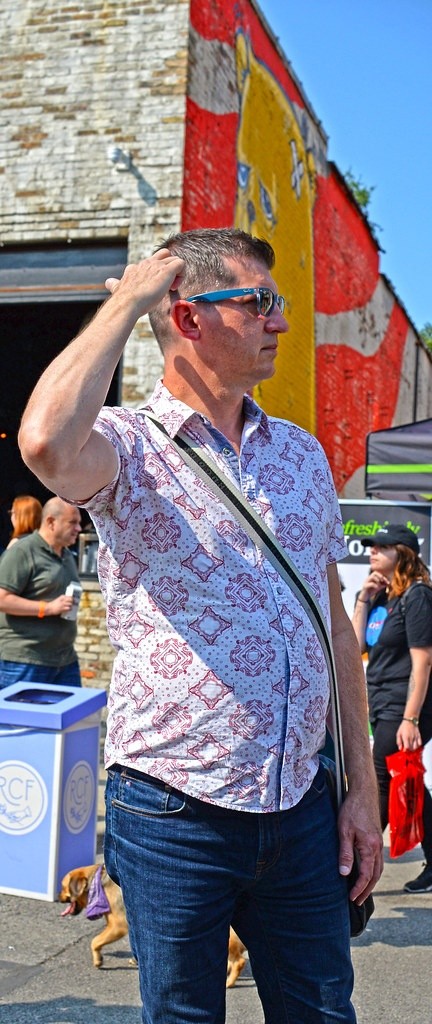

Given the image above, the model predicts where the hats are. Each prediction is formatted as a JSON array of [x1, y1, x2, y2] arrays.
[[360, 523, 419, 552]]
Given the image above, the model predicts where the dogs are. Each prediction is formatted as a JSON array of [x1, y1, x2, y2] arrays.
[[57, 863, 250, 988]]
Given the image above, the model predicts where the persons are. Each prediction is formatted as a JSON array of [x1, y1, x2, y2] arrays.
[[355, 519, 431, 893], [15, 226, 381, 1024], [1, 497, 85, 691], [7, 494, 42, 553]]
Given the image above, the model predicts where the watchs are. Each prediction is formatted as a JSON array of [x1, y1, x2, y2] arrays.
[[401, 714, 419, 726]]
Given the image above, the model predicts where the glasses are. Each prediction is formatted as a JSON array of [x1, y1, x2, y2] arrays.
[[168, 286, 285, 316]]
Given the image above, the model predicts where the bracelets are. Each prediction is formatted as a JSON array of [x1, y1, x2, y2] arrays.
[[357, 597, 369, 603], [38, 601, 46, 619]]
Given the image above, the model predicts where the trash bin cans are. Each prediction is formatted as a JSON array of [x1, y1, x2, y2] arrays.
[[0, 680, 108, 902]]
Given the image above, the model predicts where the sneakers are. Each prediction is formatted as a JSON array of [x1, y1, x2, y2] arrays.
[[403, 864, 432, 892]]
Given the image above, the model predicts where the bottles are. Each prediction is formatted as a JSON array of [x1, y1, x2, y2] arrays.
[[81, 543, 90, 574], [91, 546, 99, 574]]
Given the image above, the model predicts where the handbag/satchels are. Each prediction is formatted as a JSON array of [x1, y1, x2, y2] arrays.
[[386, 746, 423, 857], [347, 853, 376, 941]]
